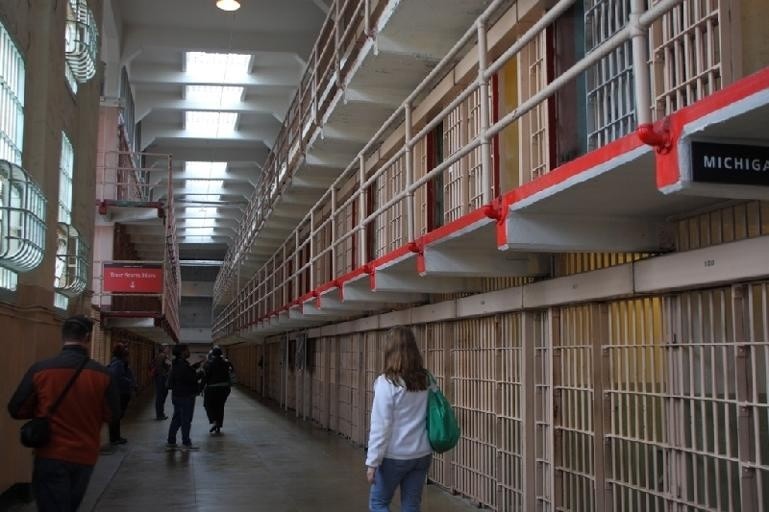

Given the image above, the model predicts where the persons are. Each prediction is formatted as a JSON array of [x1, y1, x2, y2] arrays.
[[152, 351, 171, 421], [365, 325, 441, 510], [5, 313, 111, 511], [199, 345, 237, 443], [103, 340, 131, 445], [165, 340, 208, 450]]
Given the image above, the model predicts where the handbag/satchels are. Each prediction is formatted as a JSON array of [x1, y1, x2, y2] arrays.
[[426, 375, 460, 453], [19, 417, 52, 449], [229, 367, 239, 386]]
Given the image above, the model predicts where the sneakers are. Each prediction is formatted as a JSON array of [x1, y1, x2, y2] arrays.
[[111, 414, 220, 453]]
